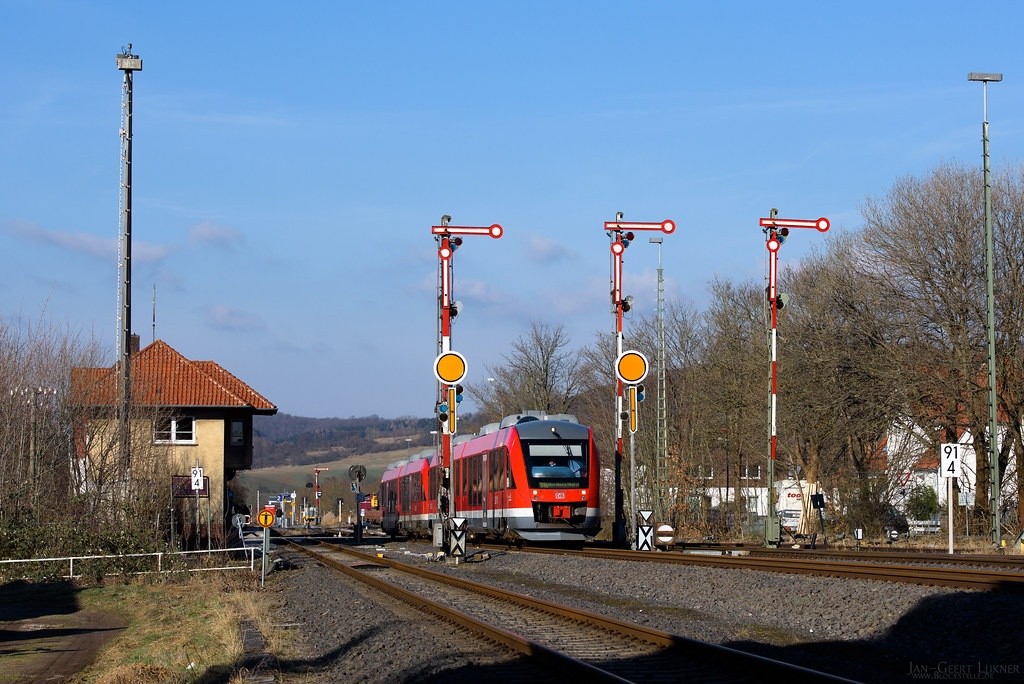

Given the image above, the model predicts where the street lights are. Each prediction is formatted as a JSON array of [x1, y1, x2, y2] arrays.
[[966, 70, 1002, 548]]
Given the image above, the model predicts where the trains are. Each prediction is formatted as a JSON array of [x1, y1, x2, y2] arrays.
[[378, 408, 603, 548]]
